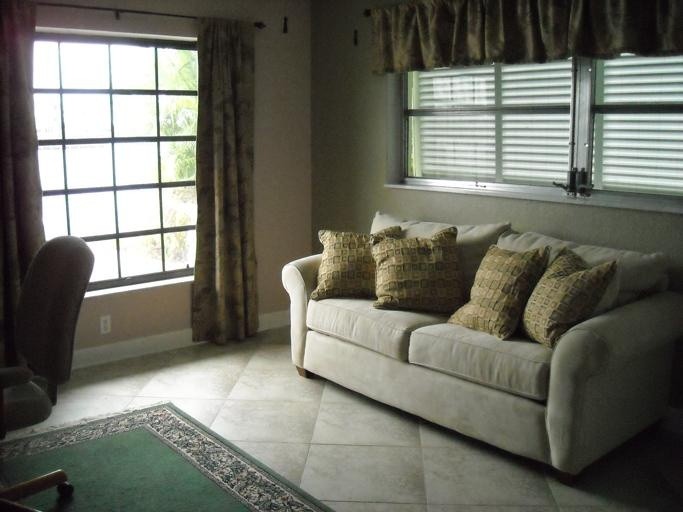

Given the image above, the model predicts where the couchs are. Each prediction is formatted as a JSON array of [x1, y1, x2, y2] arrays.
[[279, 208, 681, 484]]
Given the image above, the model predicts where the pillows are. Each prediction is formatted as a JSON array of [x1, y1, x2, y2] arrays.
[[524, 246, 614, 349], [367, 225, 464, 313], [309, 222, 403, 301], [446, 241, 553, 340]]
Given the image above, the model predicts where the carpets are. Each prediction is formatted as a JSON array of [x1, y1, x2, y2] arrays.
[[0, 402, 336, 512]]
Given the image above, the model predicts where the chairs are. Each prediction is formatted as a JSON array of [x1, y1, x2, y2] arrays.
[[0, 231, 97, 512]]
